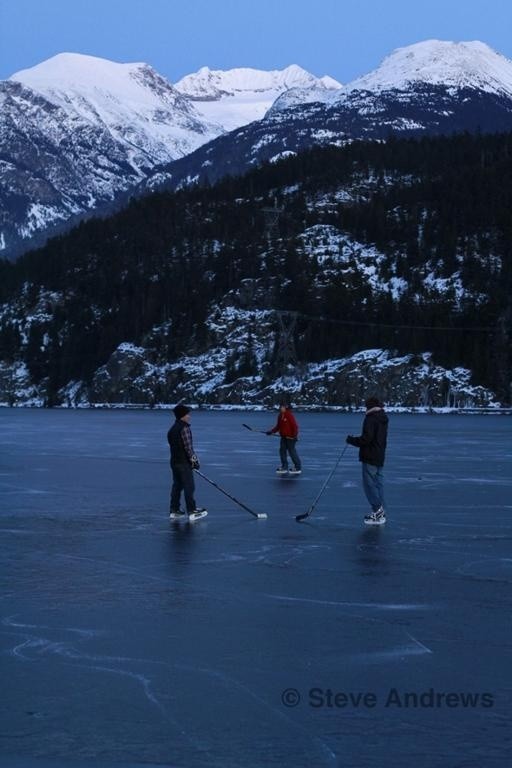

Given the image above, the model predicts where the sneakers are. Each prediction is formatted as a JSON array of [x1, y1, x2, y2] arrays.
[[187, 507, 206, 514], [277, 466, 288, 470], [170, 507, 185, 514], [365, 506, 386, 519], [289, 467, 301, 471]]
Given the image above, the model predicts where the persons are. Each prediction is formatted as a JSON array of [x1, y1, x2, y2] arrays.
[[343, 396, 390, 522], [167, 403, 207, 521], [264, 402, 302, 473]]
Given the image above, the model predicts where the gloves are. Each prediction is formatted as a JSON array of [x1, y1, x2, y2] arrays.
[[347, 435, 353, 443], [191, 454, 199, 470], [266, 430, 272, 435]]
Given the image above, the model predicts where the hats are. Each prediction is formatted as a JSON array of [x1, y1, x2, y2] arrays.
[[172, 403, 192, 419]]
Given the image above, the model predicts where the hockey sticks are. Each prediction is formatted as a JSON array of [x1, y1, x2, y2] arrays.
[[295, 432, 355, 521], [241, 422, 295, 441], [192, 466, 268, 519]]
